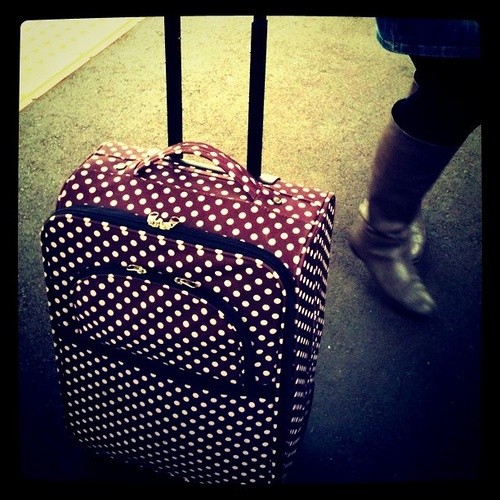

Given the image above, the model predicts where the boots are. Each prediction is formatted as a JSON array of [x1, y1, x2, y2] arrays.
[[347, 97, 461, 317]]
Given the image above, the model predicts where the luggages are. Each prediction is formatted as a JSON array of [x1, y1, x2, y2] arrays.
[[37, 17, 337, 486]]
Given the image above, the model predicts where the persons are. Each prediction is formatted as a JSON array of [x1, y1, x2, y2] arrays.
[[346, 17, 490, 320]]
